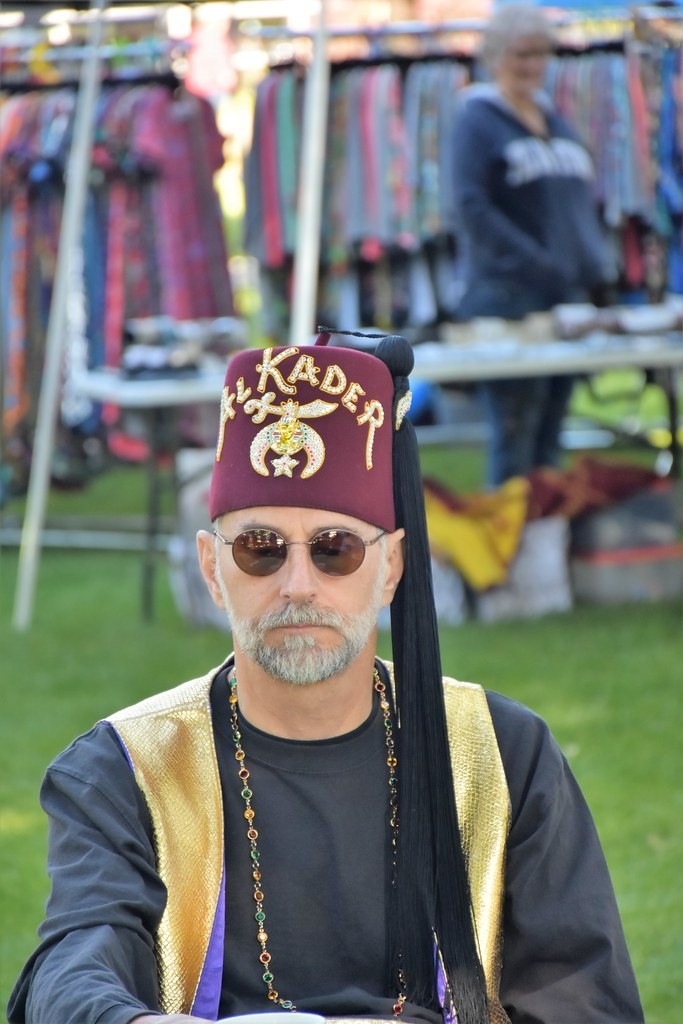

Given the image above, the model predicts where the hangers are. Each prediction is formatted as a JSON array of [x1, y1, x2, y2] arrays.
[[2, 45, 183, 92], [271, 10, 666, 72]]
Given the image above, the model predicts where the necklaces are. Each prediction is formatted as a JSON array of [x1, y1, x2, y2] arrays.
[[223, 665, 418, 1024]]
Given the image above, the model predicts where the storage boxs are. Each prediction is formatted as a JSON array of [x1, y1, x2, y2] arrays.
[[174, 515, 235, 628], [431, 488, 681, 627]]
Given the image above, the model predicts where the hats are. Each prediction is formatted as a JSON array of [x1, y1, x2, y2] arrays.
[[209, 331, 398, 529]]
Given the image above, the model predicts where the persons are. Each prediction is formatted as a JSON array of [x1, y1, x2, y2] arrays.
[[19, 342, 647, 1024], [443, 14, 612, 495]]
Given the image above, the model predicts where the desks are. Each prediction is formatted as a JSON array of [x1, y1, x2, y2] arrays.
[[78, 324, 683, 620]]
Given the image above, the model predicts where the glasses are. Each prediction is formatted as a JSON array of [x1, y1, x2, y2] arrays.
[[213, 530, 388, 575], [505, 49, 552, 61]]
